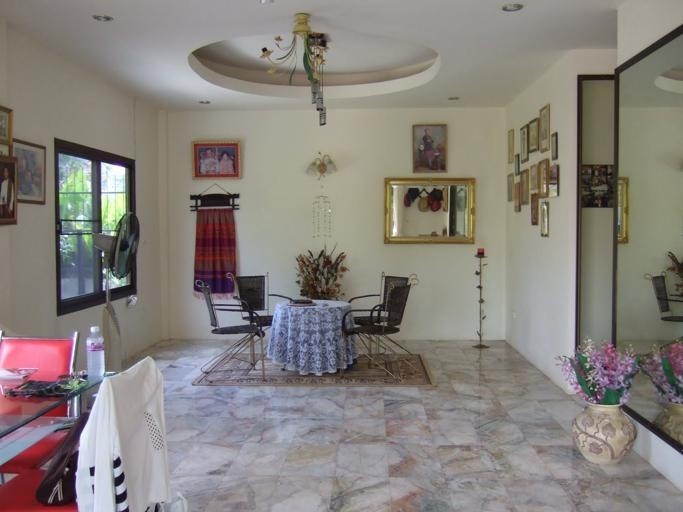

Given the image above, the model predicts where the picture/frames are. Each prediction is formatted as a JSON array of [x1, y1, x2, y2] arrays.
[[383, 177, 474, 246], [190, 140, 242, 180], [0, 106, 46, 226], [506, 104, 560, 239], [617, 175, 629, 245], [411, 123, 447, 174]]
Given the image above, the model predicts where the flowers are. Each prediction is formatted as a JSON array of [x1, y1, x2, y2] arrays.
[[294, 243, 351, 299], [636, 337, 682, 403], [668, 248, 682, 296], [555, 334, 639, 405]]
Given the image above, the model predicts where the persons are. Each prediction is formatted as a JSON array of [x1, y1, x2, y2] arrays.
[[199, 149, 219, 173], [0, 168, 15, 218], [423, 128, 436, 169], [220, 153, 234, 173]]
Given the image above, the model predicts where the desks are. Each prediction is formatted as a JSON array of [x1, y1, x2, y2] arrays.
[[0, 372, 109, 441]]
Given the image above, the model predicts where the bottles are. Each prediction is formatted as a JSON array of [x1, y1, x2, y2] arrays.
[[85, 326, 106, 377]]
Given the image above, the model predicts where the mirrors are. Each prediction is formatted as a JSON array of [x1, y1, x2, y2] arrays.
[[611, 23, 682, 455]]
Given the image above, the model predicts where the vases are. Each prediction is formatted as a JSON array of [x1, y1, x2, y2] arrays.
[[314, 300, 326, 308], [651, 401, 682, 449], [571, 397, 638, 466]]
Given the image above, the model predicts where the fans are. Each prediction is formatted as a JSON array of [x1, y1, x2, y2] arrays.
[[91, 212, 139, 372]]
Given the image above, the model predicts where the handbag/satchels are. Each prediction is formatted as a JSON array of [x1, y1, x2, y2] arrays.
[[35, 411, 90, 506]]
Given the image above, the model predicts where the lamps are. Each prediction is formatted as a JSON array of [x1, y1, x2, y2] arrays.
[[472, 248, 490, 348], [310, 185, 333, 253], [306, 155, 337, 181], [257, 14, 330, 126]]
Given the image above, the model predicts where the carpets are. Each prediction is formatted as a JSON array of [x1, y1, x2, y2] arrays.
[[190, 353, 436, 388]]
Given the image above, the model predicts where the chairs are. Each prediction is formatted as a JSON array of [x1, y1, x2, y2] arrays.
[[334, 271, 421, 383], [193, 278, 267, 383], [1, 355, 152, 511], [643, 270, 682, 324], [226, 271, 295, 373], [0, 330, 79, 485]]
